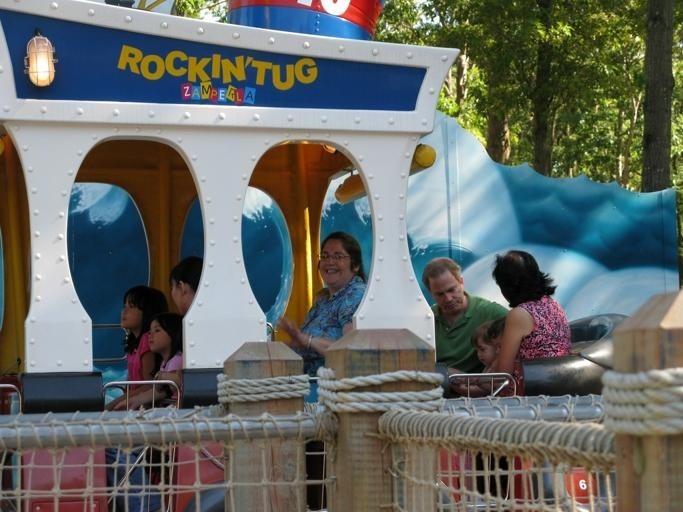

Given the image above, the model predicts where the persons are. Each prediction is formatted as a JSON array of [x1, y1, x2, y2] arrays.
[[272, 231, 367, 512], [104, 256, 203, 512], [421, 250, 572, 498]]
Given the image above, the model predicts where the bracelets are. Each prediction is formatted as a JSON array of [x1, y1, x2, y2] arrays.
[[306, 335, 312, 351]]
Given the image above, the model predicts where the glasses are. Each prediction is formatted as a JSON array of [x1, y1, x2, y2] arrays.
[[320, 253, 352, 260]]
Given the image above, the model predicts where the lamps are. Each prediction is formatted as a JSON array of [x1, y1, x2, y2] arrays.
[[24, 26, 56, 88]]
